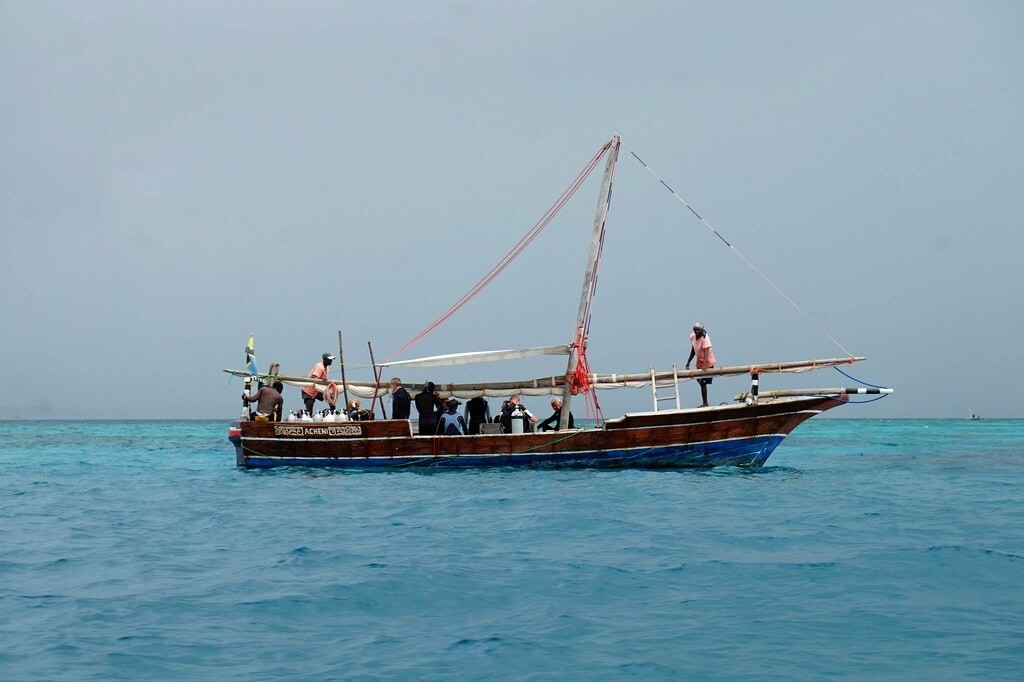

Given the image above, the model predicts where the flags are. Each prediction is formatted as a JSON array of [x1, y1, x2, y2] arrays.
[[245, 336, 257, 376]]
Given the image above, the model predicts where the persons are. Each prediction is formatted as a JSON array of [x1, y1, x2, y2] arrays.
[[436, 399, 468, 435], [415, 382, 442, 435], [685, 322, 716, 408], [301, 352, 340, 415], [390, 378, 412, 419], [537, 397, 574, 432], [494, 395, 538, 434], [434, 398, 449, 424], [464, 396, 492, 435], [242, 381, 283, 422]]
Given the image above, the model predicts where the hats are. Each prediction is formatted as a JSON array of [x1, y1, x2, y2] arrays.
[[322, 352, 336, 360]]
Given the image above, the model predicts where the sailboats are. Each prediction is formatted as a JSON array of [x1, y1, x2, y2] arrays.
[[223, 130, 894, 467], [963, 408, 980, 420]]
